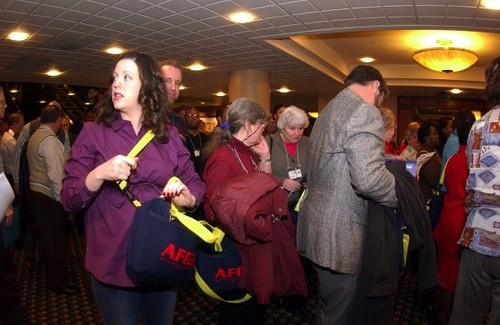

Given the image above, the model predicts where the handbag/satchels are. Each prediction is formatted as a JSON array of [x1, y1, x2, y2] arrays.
[[126, 198, 252, 304], [425, 152, 458, 232]]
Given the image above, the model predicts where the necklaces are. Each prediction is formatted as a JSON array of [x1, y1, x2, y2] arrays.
[[281, 133, 303, 179], [227, 142, 261, 174], [187, 131, 201, 157]]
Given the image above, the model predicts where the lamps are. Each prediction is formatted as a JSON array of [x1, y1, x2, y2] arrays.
[[412, 38, 478, 74]]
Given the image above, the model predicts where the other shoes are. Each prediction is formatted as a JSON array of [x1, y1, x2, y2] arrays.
[[55, 281, 80, 296]]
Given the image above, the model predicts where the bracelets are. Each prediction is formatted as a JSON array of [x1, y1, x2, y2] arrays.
[[258, 156, 272, 163]]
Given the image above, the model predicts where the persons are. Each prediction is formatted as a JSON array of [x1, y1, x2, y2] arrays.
[[12, 101, 72, 275], [172, 102, 232, 161], [378, 108, 477, 255], [179, 106, 211, 182], [0, 84, 24, 325], [158, 59, 190, 152], [0, 117, 84, 153], [60, 51, 208, 325], [447, 57, 500, 325], [202, 97, 272, 325], [264, 105, 317, 324], [422, 120, 475, 325], [262, 103, 317, 138], [27, 104, 80, 296], [1, 113, 25, 250], [297, 65, 399, 325]]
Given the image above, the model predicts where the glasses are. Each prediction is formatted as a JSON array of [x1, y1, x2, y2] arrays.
[[188, 114, 199, 117]]
